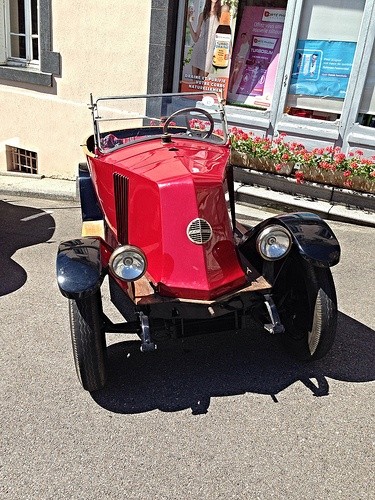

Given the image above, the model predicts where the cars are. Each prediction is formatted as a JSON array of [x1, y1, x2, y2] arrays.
[[55, 91, 343, 392]]
